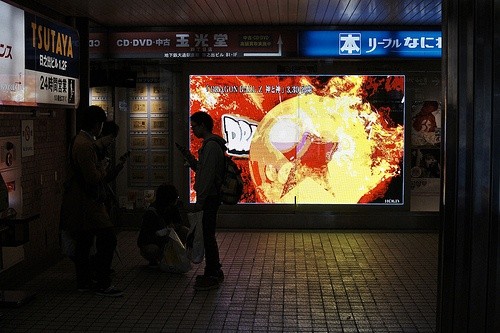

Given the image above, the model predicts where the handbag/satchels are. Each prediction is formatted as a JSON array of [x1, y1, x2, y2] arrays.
[[185, 211, 205, 263], [145, 227, 194, 273], [60, 183, 97, 233]]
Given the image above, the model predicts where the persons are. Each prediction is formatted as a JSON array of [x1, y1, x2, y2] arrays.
[[93, 118, 124, 235], [60, 106, 126, 296], [175, 110, 224, 291], [135, 184, 203, 264]]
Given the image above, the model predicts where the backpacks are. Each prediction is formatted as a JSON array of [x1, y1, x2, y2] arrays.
[[202, 138, 243, 204]]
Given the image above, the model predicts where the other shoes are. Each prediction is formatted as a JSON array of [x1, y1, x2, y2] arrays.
[[77, 280, 98, 292], [96, 287, 124, 297]]
